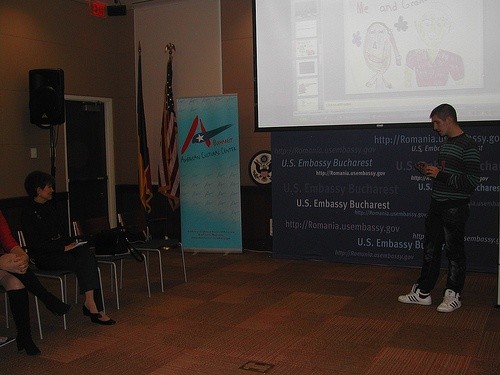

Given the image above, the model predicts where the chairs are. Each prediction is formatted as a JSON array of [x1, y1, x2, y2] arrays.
[[0, 213, 188, 339]]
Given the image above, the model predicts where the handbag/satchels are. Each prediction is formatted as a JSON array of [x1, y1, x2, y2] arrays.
[[95, 227, 128, 255]]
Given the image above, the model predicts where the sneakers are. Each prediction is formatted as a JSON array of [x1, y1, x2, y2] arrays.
[[437, 288, 461, 312], [397, 283, 431, 305]]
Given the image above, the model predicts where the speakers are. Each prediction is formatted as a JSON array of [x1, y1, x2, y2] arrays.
[[28, 69, 66, 127]]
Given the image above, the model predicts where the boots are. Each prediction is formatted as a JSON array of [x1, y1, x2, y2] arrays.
[[8, 270, 71, 316], [6, 288, 41, 355]]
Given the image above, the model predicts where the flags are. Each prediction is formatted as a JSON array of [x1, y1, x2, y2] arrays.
[[159, 57, 180, 200], [137, 55, 153, 209]]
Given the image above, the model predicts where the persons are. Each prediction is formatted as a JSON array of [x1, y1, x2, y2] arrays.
[[0, 210, 71, 356], [24, 171, 116, 325], [399, 103, 482, 313]]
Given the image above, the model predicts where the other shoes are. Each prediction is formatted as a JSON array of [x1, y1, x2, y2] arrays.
[[82, 304, 102, 318], [91, 316, 116, 325]]
